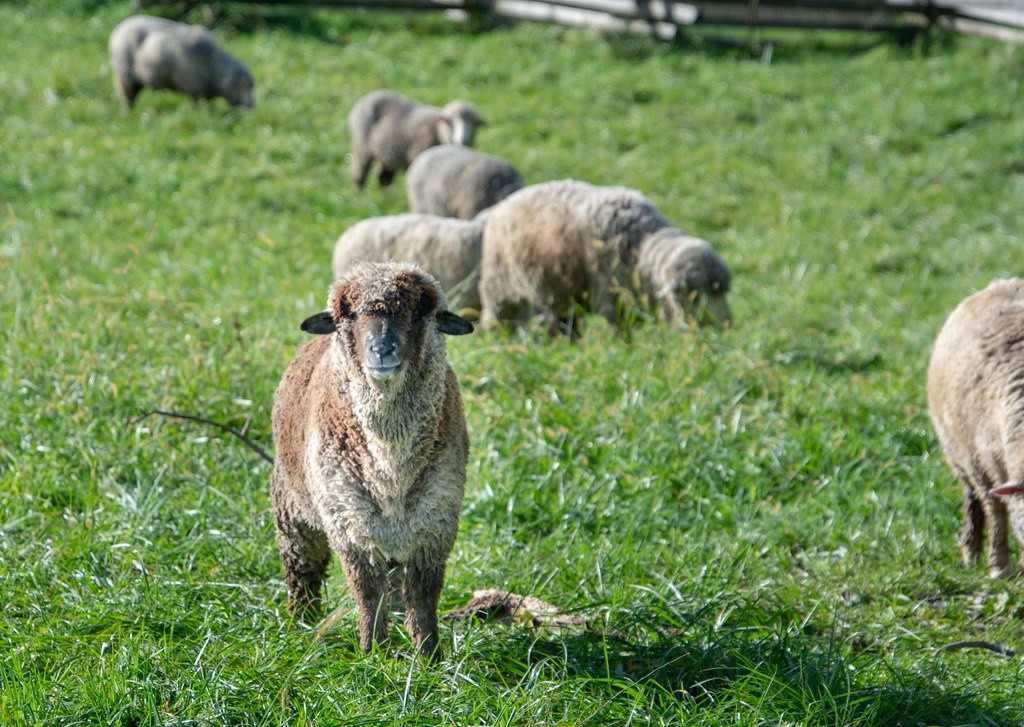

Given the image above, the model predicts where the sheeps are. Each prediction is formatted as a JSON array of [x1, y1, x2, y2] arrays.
[[333, 179, 733, 341], [270, 263, 474, 661], [344, 89, 521, 217], [927, 276, 1024, 577], [108, 16, 253, 117]]
